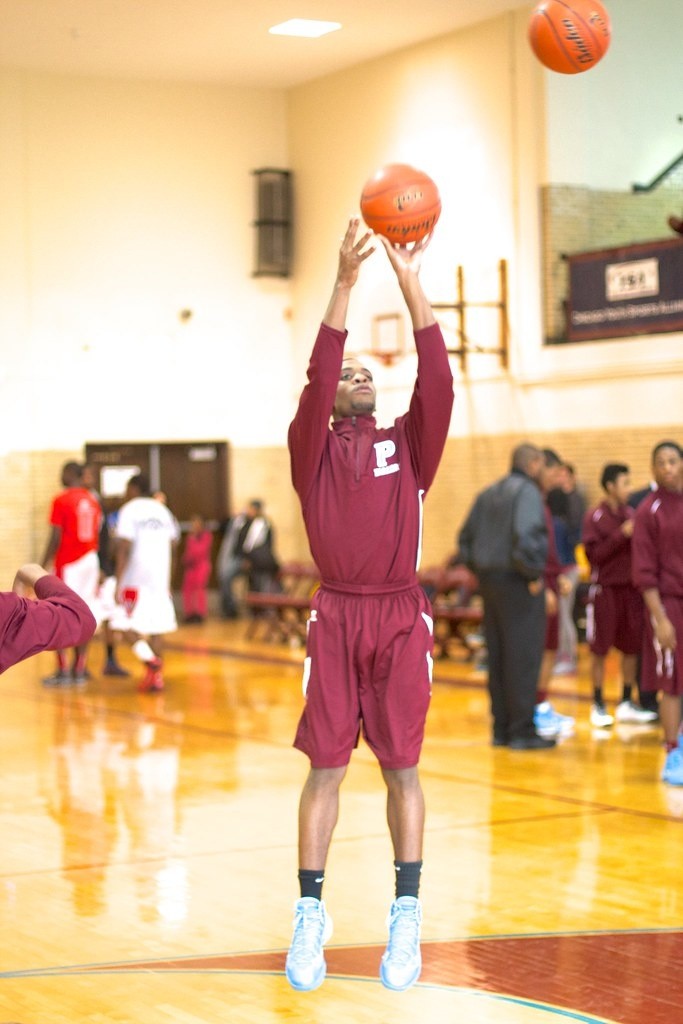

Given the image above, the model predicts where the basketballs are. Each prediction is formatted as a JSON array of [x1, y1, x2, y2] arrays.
[[357, 162, 442, 244], [526, 0, 613, 76]]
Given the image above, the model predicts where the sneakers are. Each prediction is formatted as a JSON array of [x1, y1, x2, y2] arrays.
[[379, 896, 422, 991], [286, 896, 334, 990]]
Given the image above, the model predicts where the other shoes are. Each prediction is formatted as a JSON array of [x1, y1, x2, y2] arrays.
[[492, 737, 511, 745], [661, 735, 683, 785], [532, 701, 574, 735], [43, 671, 85, 688], [103, 662, 129, 676], [591, 709, 615, 726], [617, 702, 658, 723], [511, 736, 556, 751], [137, 657, 164, 692]]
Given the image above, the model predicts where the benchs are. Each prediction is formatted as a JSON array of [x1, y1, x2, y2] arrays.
[[430, 605, 489, 628], [242, 592, 312, 649]]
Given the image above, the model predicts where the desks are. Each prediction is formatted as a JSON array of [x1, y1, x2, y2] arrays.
[[422, 567, 487, 670], [265, 562, 322, 589]]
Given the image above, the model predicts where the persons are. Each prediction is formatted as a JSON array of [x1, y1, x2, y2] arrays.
[[38, 462, 282, 693], [0, 563, 97, 675], [458, 440, 683, 788], [285, 217, 455, 991]]
